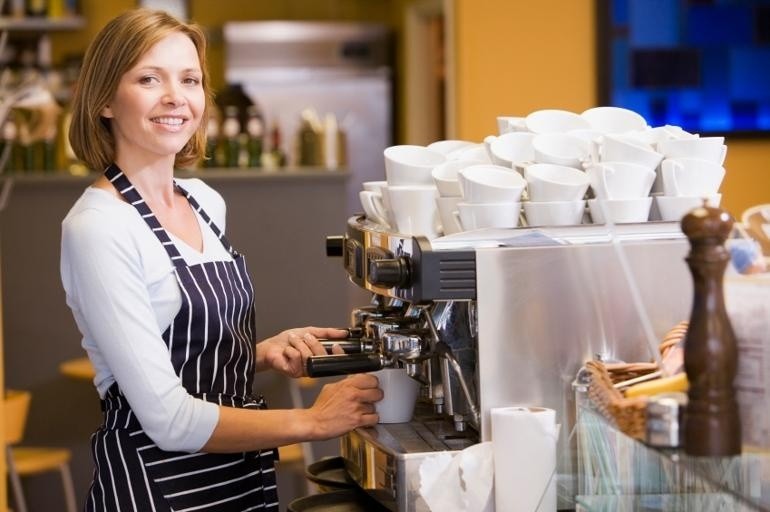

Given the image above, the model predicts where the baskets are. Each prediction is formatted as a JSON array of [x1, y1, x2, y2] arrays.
[[585, 320, 689, 440]]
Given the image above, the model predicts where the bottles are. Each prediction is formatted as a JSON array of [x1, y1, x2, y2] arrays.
[[201, 102, 267, 168]]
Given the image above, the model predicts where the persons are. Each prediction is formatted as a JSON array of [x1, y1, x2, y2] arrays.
[[57, 3, 387, 511]]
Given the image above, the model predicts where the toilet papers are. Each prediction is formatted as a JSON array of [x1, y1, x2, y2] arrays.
[[490, 407, 560, 512]]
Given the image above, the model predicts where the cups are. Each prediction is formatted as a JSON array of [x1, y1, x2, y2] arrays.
[[356, 105, 729, 240]]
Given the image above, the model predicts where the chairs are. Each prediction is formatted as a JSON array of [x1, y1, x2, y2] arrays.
[[8, 447, 79, 511], [273, 374, 319, 511], [1, 388, 30, 512]]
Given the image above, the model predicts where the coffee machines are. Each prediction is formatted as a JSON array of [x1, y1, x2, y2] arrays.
[[306, 214, 734, 510]]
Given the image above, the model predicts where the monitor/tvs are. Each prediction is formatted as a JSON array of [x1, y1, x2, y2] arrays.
[[595, 1, 769, 141]]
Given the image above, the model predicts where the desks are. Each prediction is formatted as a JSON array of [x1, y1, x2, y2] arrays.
[[61, 358, 97, 379]]
